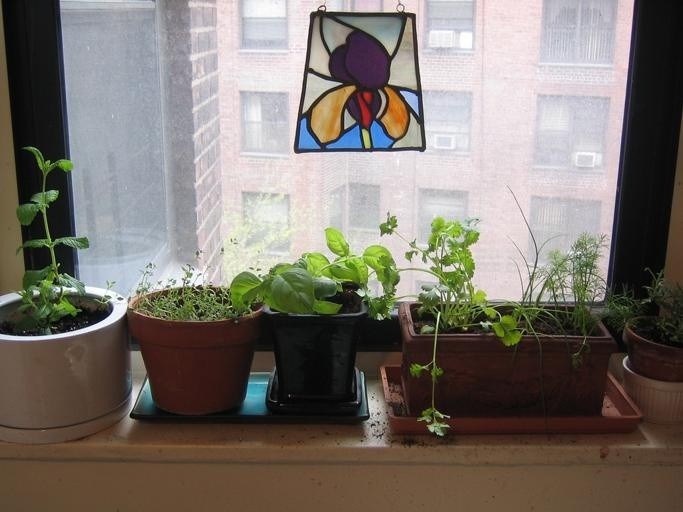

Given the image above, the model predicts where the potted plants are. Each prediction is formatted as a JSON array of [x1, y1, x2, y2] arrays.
[[369, 184, 643, 435], [621, 267, 683, 381], [230, 228, 396, 416], [0, 147, 137, 444], [128, 247, 263, 417]]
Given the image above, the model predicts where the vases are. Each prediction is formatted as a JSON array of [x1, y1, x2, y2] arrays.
[[622, 354, 683, 424]]
